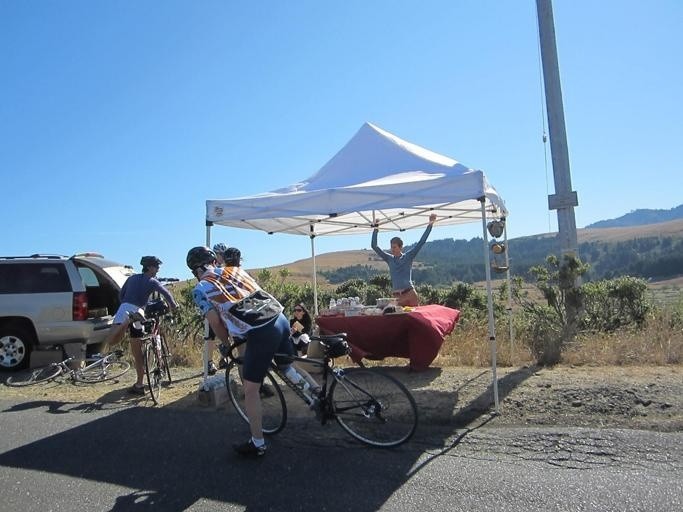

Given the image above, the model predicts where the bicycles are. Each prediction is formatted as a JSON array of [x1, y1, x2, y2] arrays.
[[6, 343, 131, 386], [217, 333, 419, 448], [138, 297, 178, 406]]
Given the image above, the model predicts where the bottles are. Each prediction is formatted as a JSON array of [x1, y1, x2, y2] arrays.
[[156, 335, 161, 351], [283, 364, 310, 392]]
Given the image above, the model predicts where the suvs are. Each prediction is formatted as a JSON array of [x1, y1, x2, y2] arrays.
[[0, 256, 138, 371]]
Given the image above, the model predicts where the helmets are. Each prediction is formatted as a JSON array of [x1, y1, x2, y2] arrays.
[[186, 246, 218, 271], [140, 256, 162, 269], [224, 248, 242, 266], [213, 243, 227, 257]]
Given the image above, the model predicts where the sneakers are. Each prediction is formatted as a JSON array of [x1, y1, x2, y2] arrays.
[[219, 358, 234, 369], [208, 359, 217, 373], [128, 310, 145, 322], [132, 383, 145, 395], [308, 386, 323, 411], [232, 438, 267, 458]]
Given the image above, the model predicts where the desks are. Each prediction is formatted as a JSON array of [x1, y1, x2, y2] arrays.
[[313, 303, 442, 371]]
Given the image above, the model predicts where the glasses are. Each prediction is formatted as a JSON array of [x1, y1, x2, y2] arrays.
[[294, 309, 301, 312]]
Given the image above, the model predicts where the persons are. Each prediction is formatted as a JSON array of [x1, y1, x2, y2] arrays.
[[187, 242, 336, 457], [370, 213, 437, 306], [106, 254, 179, 395]]
